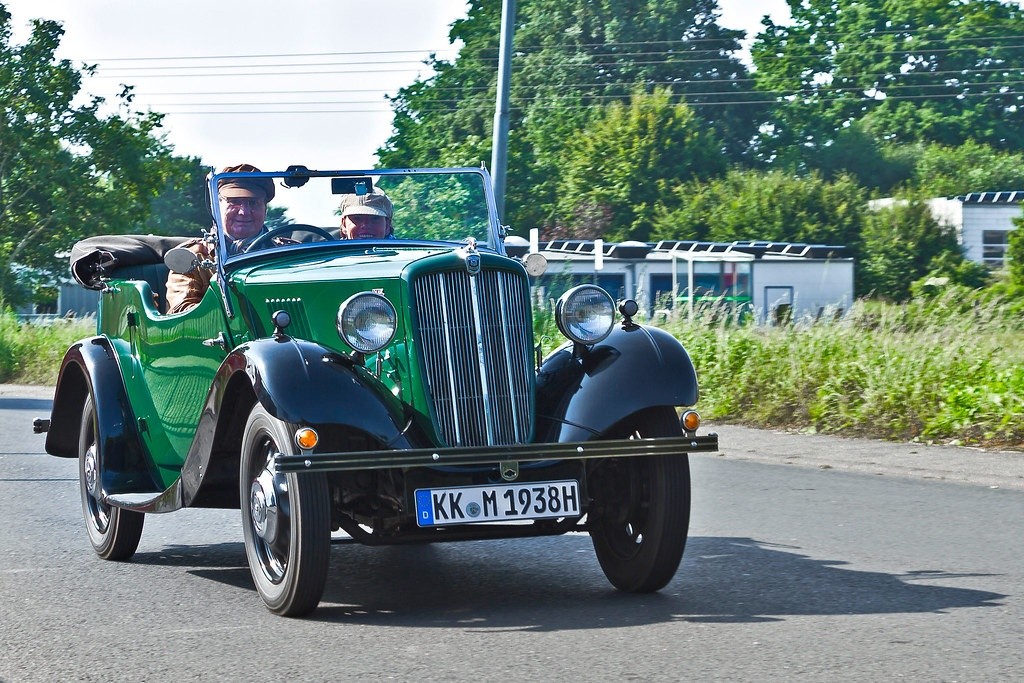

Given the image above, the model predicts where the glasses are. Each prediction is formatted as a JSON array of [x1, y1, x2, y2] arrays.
[[219, 195, 264, 210]]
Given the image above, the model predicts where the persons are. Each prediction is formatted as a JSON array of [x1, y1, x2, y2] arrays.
[[166, 163, 304, 314], [339, 186, 394, 241]]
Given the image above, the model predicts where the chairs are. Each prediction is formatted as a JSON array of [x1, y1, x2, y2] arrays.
[[118, 263, 167, 311]]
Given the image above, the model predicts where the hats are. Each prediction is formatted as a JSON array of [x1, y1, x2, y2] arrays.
[[339, 186, 394, 220], [206, 163, 275, 202]]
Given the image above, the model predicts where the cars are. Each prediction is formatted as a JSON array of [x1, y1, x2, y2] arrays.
[[32, 166, 720, 615]]
